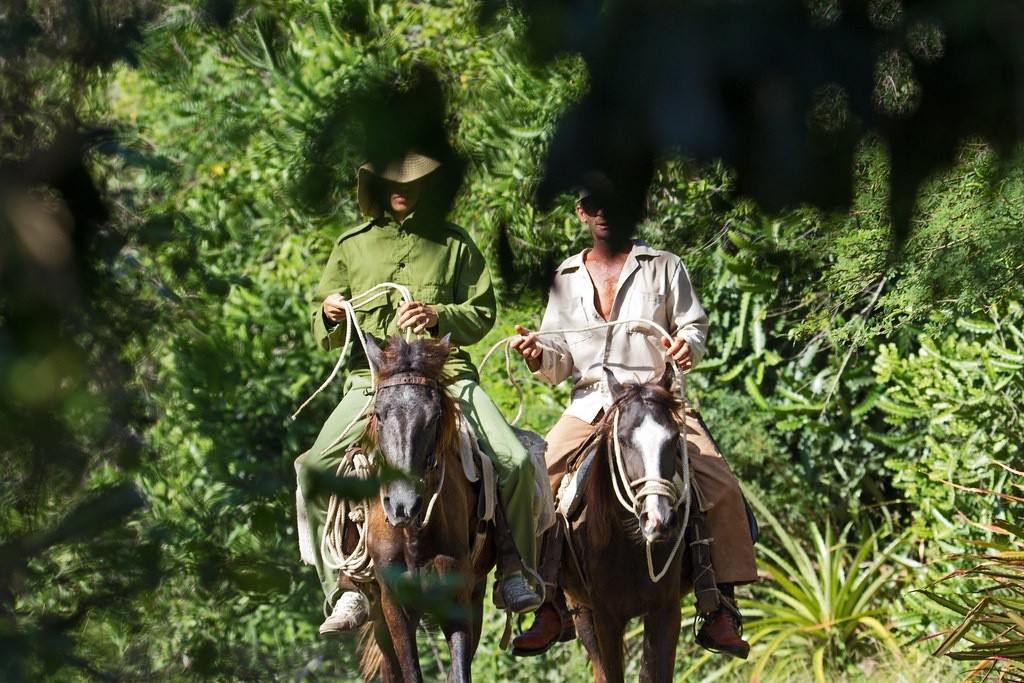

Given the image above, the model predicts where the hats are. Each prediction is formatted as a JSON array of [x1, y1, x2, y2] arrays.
[[576, 189, 590, 204], [357, 147, 442, 216]]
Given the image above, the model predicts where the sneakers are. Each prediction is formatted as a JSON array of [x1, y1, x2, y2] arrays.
[[496, 574, 542, 614], [319, 590, 368, 634]]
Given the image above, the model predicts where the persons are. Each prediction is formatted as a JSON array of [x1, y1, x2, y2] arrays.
[[297, 156, 543, 636], [509, 192, 757, 660]]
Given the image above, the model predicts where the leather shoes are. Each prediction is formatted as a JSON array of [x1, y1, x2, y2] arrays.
[[513, 588, 576, 651], [694, 610, 749, 659]]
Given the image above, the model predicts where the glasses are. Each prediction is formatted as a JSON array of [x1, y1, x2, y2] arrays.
[[580, 193, 600, 218]]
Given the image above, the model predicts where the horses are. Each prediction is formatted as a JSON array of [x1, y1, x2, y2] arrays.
[[334, 332, 502, 682], [540, 361, 704, 683]]
